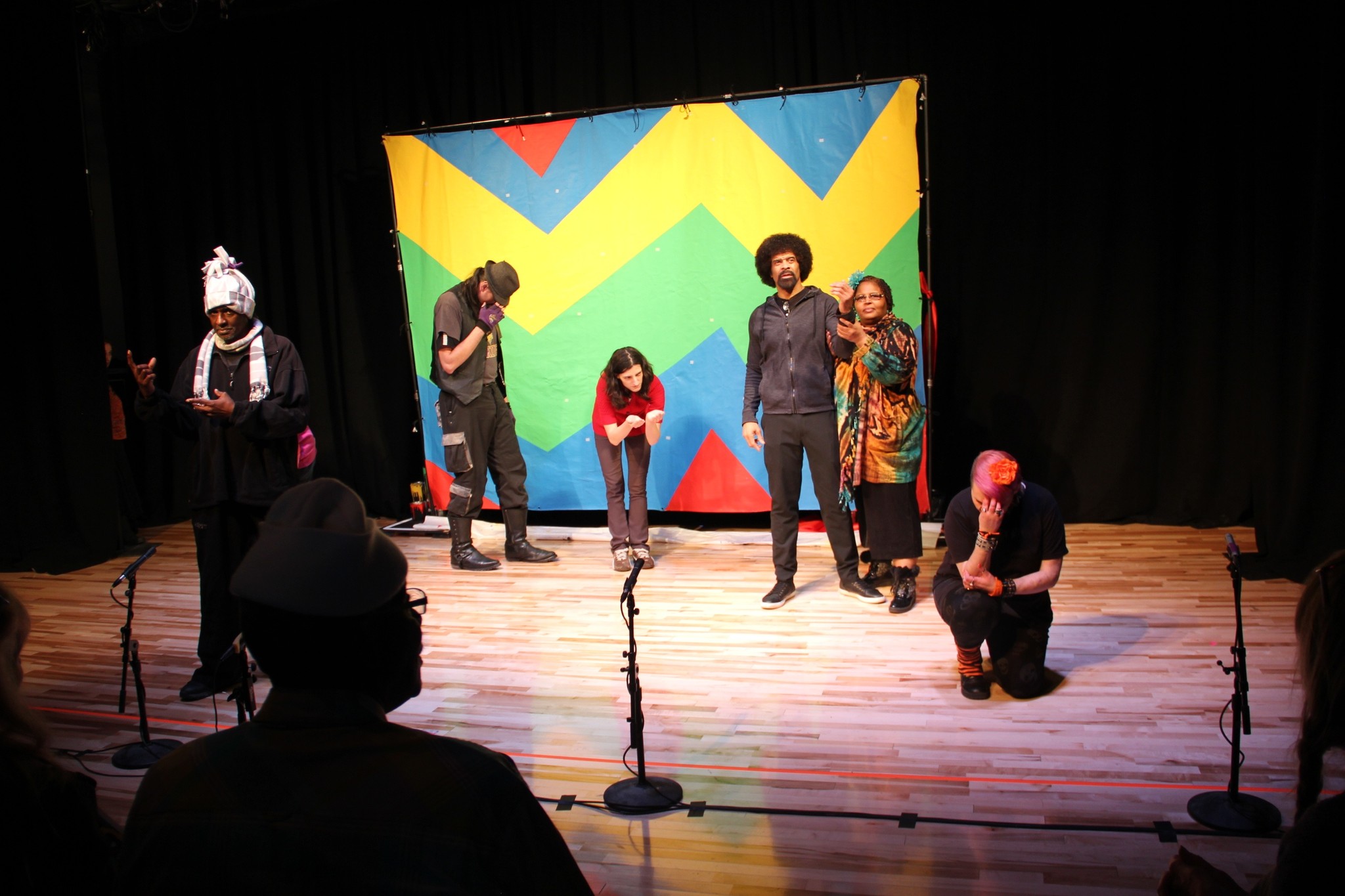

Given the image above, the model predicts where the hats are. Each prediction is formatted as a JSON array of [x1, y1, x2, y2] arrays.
[[229, 477, 407, 654], [200, 244, 255, 319], [485, 260, 520, 307]]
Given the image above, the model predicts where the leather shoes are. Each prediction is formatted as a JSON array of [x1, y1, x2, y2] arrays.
[[960, 673, 991, 699]]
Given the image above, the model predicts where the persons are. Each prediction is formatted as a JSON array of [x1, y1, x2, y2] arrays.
[[428, 261, 558, 570], [827, 269, 930, 614], [1158, 551, 1345, 896], [742, 232, 886, 609], [105, 338, 149, 555], [128, 244, 310, 702], [933, 449, 1069, 700], [0, 580, 156, 895], [592, 347, 665, 572], [106, 478, 592, 895]]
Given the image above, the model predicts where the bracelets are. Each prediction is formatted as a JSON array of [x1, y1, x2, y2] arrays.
[[975, 531, 1000, 551], [988, 576, 1016, 598]]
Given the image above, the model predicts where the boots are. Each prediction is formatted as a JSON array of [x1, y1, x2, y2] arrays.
[[502, 507, 557, 563], [888, 565, 919, 612], [447, 516, 501, 571], [860, 550, 893, 586]]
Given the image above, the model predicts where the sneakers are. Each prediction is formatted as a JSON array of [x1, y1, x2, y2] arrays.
[[612, 547, 631, 571], [762, 578, 795, 609], [839, 579, 886, 602], [633, 548, 654, 568]]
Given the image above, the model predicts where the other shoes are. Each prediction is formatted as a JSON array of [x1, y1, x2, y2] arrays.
[[181, 652, 246, 698]]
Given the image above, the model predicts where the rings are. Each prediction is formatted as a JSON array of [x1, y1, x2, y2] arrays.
[[970, 576, 974, 586], [982, 568, 986, 573], [982, 507, 987, 513], [996, 509, 1001, 513], [967, 586, 970, 590]]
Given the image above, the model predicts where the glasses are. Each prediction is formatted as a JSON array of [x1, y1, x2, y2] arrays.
[[853, 293, 884, 301], [391, 588, 428, 615]]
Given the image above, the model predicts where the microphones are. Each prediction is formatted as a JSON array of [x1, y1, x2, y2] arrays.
[[1224, 533, 1239, 567], [111, 547, 157, 588], [620, 558, 645, 602]]
